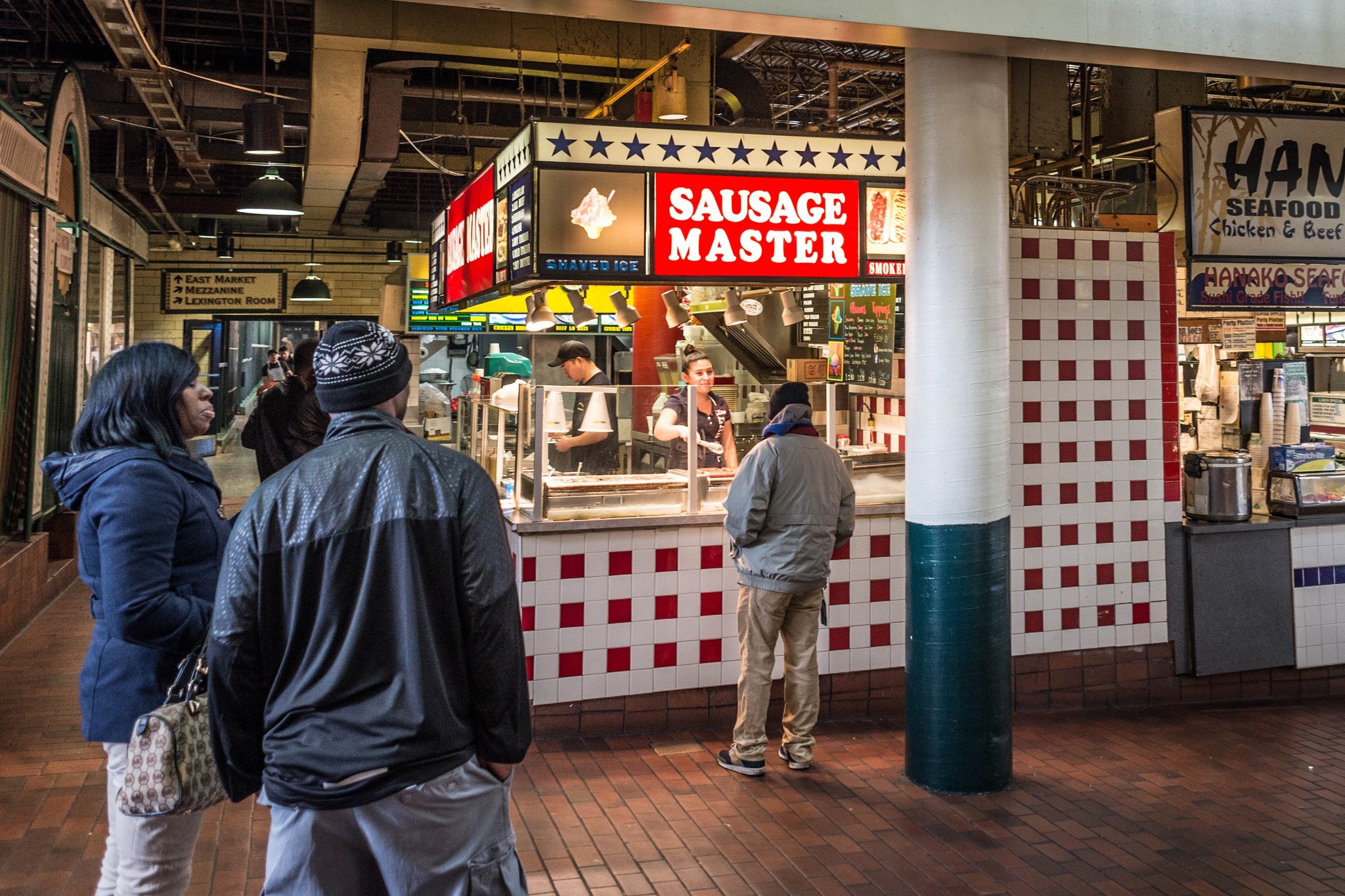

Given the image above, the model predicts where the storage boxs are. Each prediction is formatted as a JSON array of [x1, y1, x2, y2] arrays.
[[786, 358, 827, 383], [422, 416, 451, 441]]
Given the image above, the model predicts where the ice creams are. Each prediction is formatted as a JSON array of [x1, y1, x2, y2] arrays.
[[831, 353, 839, 372], [831, 283, 841, 296], [831, 306, 842, 334]]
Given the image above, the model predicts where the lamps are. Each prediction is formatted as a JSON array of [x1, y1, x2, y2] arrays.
[[196, 218, 234, 259], [235, 166, 305, 216], [609, 286, 641, 329], [242, 0, 285, 156], [577, 392, 614, 433], [22, 76, 45, 107], [567, 285, 598, 328], [724, 287, 748, 326], [658, 55, 688, 121], [661, 286, 690, 329], [290, 239, 333, 301], [543, 391, 570, 433], [780, 287, 805, 327], [267, 214, 292, 233], [386, 240, 403, 262], [525, 286, 555, 332]]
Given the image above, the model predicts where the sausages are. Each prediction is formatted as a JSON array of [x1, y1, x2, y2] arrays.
[[697, 467, 737, 478]]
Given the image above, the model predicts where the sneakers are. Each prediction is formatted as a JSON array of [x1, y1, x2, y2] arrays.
[[716, 743, 765, 775], [779, 744, 810, 768]]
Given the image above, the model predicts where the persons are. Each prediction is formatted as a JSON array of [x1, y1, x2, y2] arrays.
[[654, 343, 739, 473], [256, 337, 294, 396], [241, 338, 332, 484], [547, 340, 620, 475], [39, 341, 232, 896], [207, 320, 532, 896], [716, 381, 855, 776]]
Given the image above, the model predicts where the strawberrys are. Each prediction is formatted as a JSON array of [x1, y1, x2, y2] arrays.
[[829, 364, 841, 376]]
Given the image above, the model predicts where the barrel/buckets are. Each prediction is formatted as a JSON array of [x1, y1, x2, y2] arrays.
[[730, 411, 745, 424]]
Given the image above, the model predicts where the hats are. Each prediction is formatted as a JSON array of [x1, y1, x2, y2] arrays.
[[312, 320, 413, 413], [548, 340, 592, 367], [768, 382, 812, 421]]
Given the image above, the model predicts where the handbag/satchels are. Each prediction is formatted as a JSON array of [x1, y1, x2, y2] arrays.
[[115, 629, 229, 817]]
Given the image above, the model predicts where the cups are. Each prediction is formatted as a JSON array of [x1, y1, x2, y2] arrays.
[[475, 369, 484, 377], [1247, 367, 1302, 513], [1253, 342, 1296, 360]]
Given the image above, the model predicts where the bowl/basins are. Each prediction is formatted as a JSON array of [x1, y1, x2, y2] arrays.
[[419, 373, 450, 383], [683, 325, 718, 341]]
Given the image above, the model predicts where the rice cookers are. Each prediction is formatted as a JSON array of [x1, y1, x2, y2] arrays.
[[1183, 448, 1252, 522]]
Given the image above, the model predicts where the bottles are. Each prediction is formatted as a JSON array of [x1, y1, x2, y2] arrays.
[[746, 402, 769, 423], [485, 448, 497, 482], [837, 434, 851, 447]]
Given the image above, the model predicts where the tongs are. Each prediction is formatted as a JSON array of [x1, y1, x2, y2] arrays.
[[697, 440, 725, 455]]
